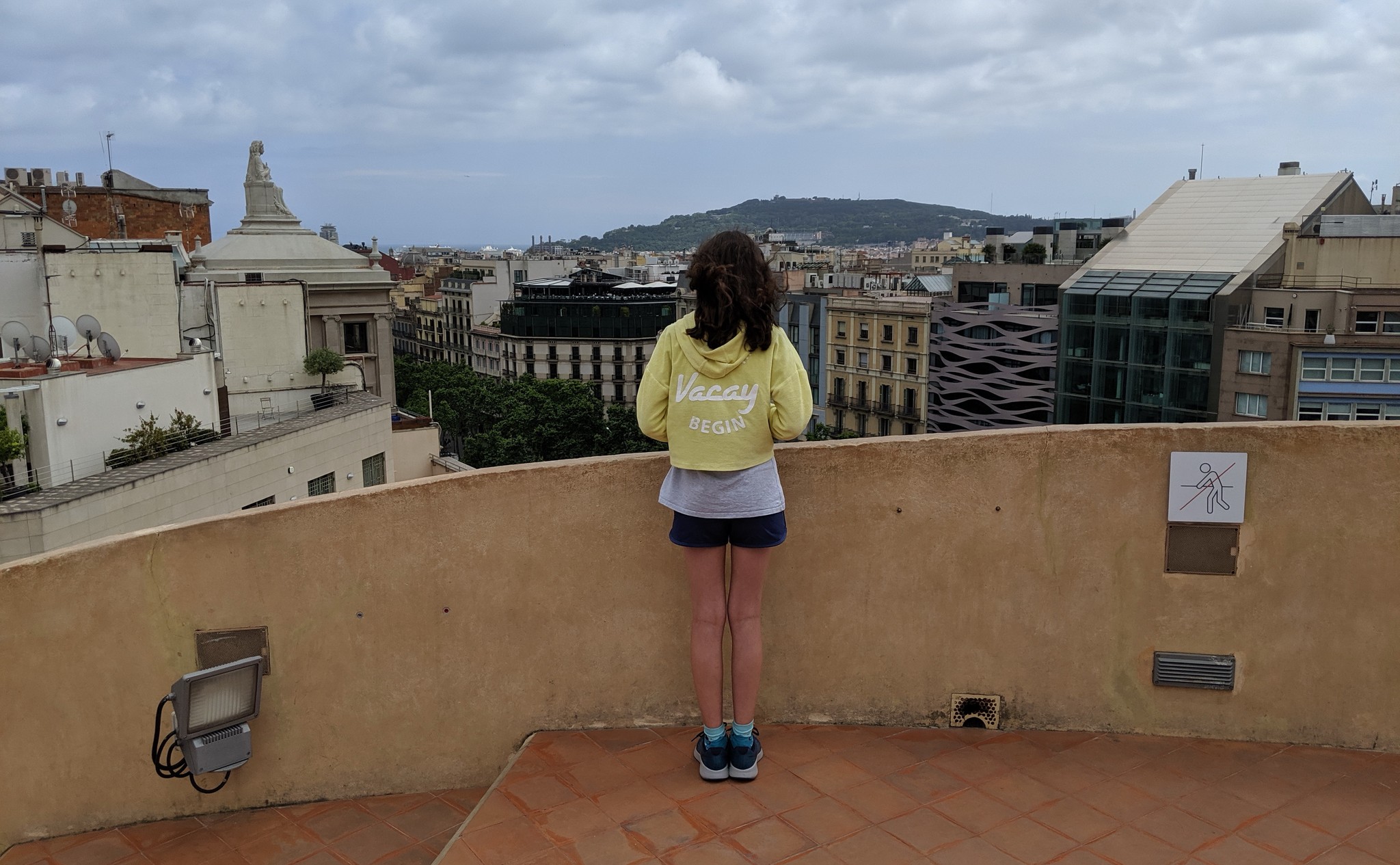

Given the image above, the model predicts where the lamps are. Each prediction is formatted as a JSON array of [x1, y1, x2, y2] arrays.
[[151, 655, 264, 794], [223, 367, 232, 374]]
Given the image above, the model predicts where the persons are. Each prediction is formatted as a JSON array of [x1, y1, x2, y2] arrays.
[[636, 230, 813, 783], [245, 138, 290, 216]]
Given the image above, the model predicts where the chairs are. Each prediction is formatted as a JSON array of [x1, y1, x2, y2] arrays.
[[259, 397, 276, 420]]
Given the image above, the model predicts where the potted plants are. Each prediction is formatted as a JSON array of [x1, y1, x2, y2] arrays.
[[1022, 243, 1048, 264], [1323, 323, 1336, 345], [1052, 242, 1060, 260], [302, 346, 344, 411], [982, 244, 996, 262]]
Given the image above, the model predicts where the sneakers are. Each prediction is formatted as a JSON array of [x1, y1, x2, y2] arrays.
[[691, 722, 729, 779], [726, 727, 763, 779]]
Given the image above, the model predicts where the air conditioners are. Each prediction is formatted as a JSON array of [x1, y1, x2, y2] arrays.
[[806, 273, 909, 290], [75, 172, 85, 186], [56, 171, 66, 186], [4, 167, 28, 186], [581, 274, 597, 282], [511, 255, 572, 260], [30, 168, 53, 186]]
